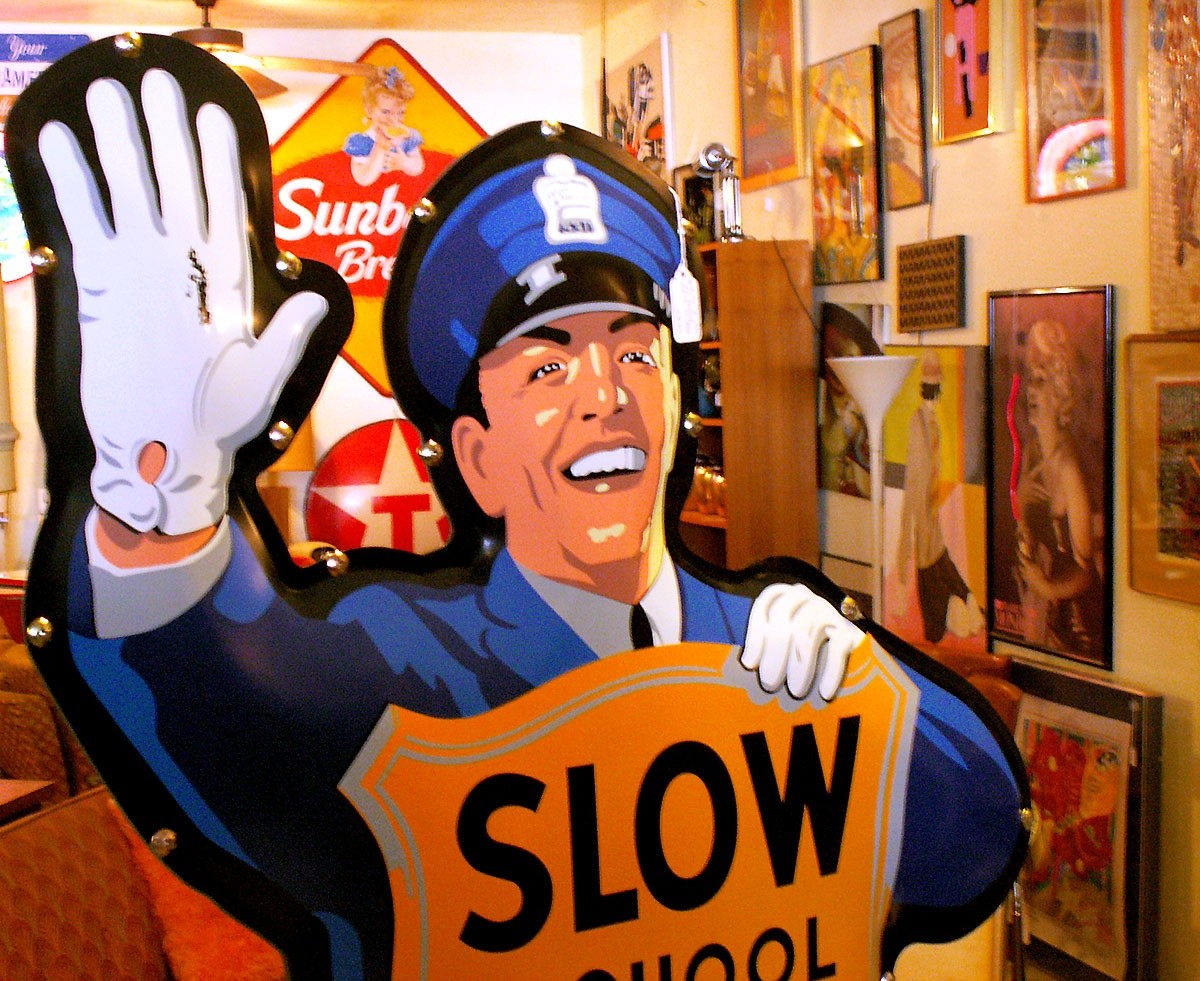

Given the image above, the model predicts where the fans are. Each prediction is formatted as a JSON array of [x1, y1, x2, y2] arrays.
[[169, 0, 375, 103]]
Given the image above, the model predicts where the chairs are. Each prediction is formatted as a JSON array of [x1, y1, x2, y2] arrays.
[[0, 637, 296, 981]]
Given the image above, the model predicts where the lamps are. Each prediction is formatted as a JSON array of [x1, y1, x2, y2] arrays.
[[823, 355, 916, 627]]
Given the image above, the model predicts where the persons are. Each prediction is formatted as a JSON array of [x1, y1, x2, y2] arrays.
[[1012, 318, 1102, 653]]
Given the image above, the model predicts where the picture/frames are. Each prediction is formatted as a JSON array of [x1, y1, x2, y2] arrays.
[[995, 653, 1163, 981], [1125, 332, 1199, 606], [984, 281, 1117, 671], [803, 44, 885, 286], [730, 0, 806, 196], [877, 0, 1127, 212]]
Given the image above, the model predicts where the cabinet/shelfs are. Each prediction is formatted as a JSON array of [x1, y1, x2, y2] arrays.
[[676, 236, 818, 574]]
